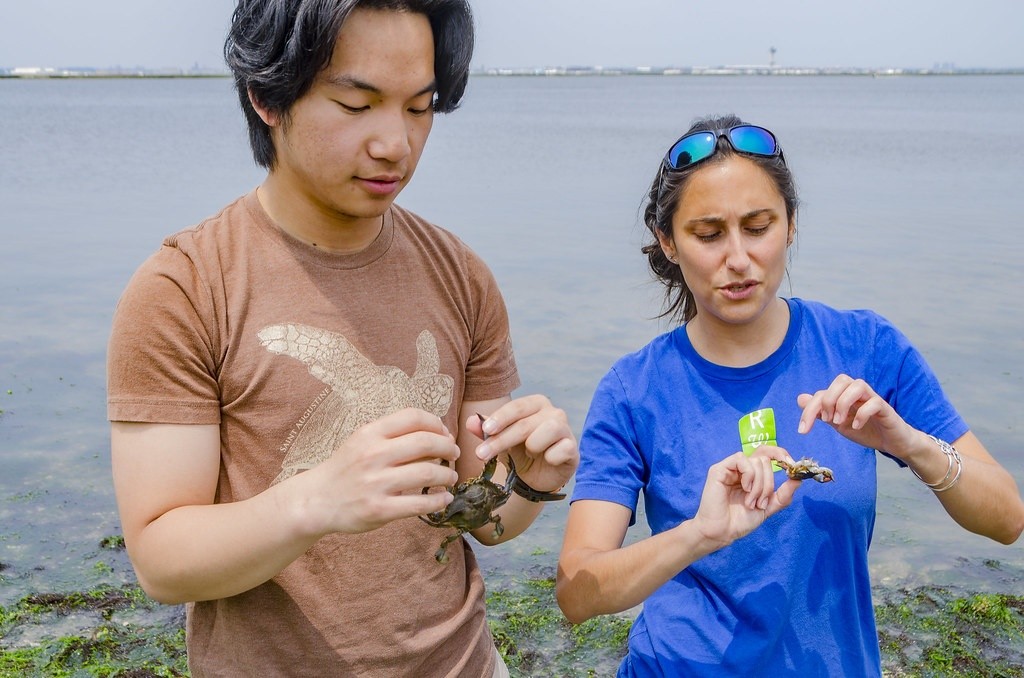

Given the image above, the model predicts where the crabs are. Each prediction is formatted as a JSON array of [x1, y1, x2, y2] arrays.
[[417, 412, 518, 564], [775, 455, 835, 484]]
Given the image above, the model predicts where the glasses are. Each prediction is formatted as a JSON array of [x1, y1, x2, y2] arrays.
[[662, 124, 786, 174]]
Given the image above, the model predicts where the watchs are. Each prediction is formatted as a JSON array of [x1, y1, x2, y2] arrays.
[[512, 472, 567, 503]]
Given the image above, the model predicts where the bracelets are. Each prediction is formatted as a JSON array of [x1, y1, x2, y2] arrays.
[[909, 434, 963, 491]]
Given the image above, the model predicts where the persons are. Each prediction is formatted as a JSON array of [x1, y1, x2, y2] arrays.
[[108, 0, 581, 678], [553, 111, 1023, 678]]
